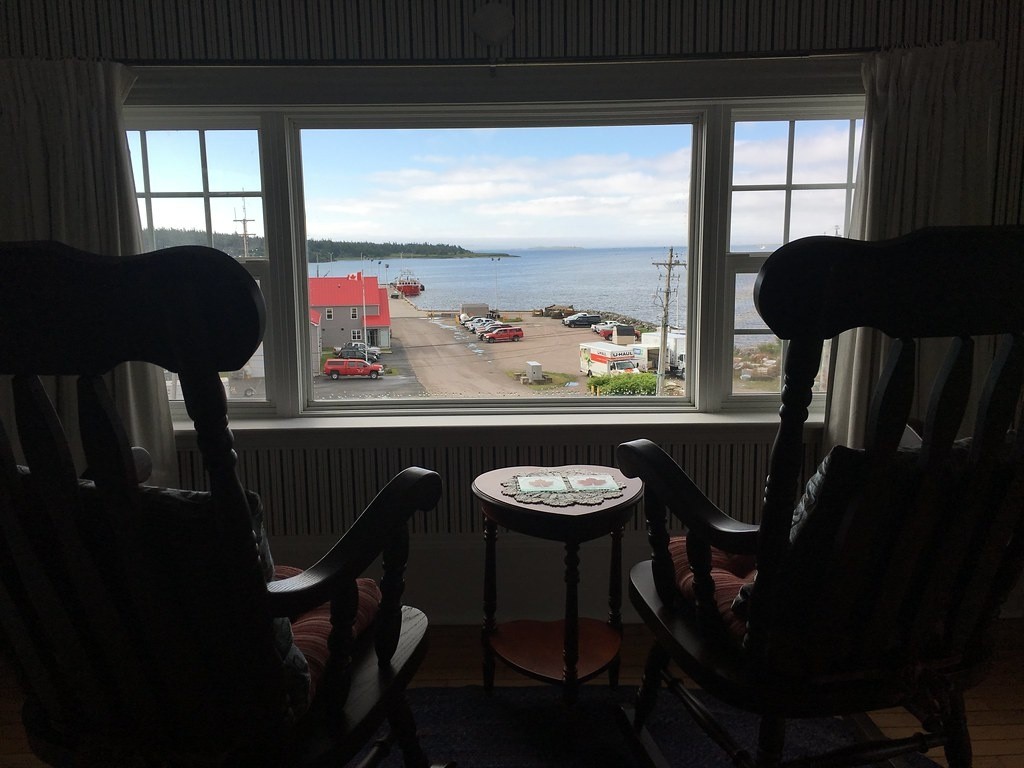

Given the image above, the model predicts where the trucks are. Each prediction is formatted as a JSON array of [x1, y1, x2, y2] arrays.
[[577, 340, 638, 381], [625, 328, 689, 377]]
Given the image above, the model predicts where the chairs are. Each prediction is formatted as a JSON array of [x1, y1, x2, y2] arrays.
[[614, 222, 1024, 768], [0, 236, 461, 768]]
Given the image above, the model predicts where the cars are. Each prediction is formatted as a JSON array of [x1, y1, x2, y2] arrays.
[[563, 311, 639, 339], [331, 339, 382, 361], [462, 315, 512, 339]]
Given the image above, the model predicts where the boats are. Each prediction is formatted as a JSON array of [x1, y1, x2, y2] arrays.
[[391, 267, 421, 295]]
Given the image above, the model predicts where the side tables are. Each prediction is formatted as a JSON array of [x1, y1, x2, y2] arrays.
[[469, 462, 649, 707]]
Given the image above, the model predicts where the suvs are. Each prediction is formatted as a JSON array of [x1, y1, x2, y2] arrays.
[[324, 359, 385, 381], [482, 327, 523, 342]]
